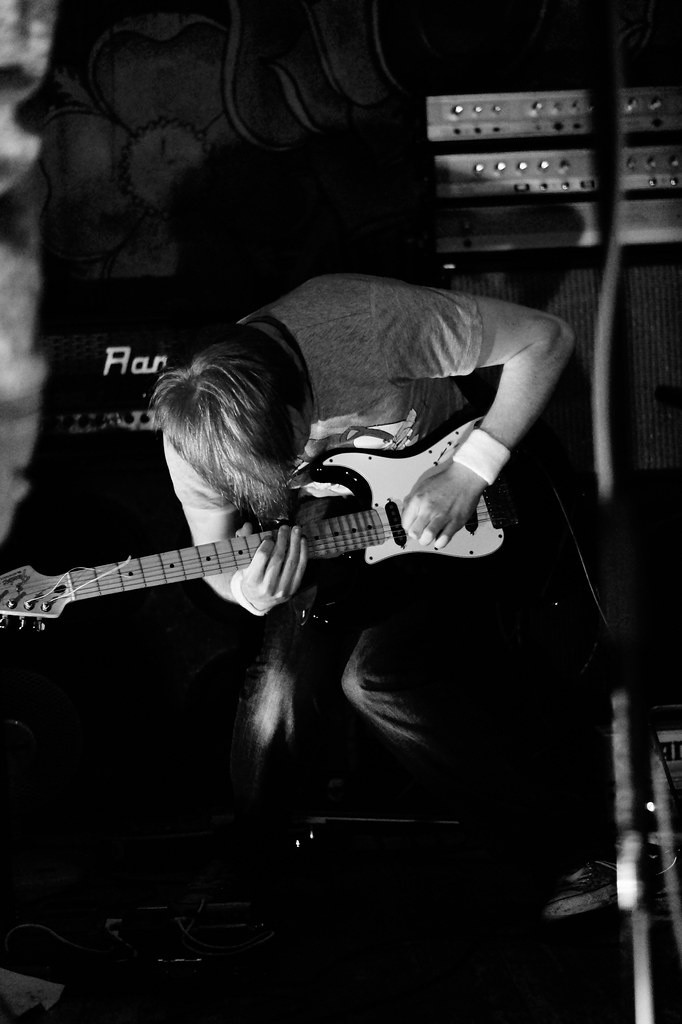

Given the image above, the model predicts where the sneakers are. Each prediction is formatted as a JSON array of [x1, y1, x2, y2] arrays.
[[169, 863, 252, 911], [542, 858, 617, 920]]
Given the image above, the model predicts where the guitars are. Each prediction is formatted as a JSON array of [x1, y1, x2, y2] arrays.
[[0, 408, 508, 634]]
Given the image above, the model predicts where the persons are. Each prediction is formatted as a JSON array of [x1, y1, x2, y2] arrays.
[[154, 274, 617, 919]]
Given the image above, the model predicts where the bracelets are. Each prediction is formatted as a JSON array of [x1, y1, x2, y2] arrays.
[[452, 429, 511, 485], [230, 570, 272, 616]]
